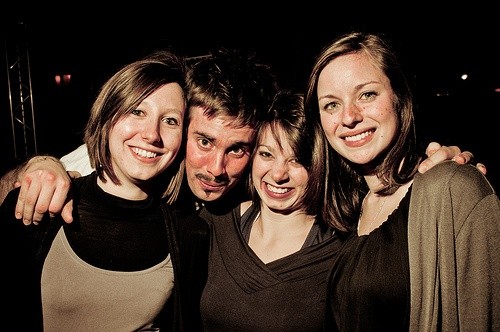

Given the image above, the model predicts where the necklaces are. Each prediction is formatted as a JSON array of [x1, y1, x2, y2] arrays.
[[358, 187, 388, 231]]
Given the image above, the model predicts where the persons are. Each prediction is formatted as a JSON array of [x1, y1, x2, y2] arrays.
[[0, 58, 211, 332], [0, 46, 487, 226], [196, 89, 370, 332], [299, 32, 500, 332]]
